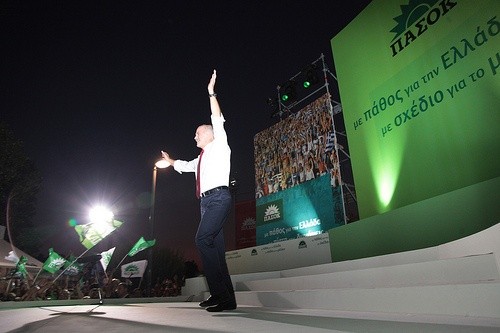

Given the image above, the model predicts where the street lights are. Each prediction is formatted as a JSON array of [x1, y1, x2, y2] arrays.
[[146, 158, 172, 297]]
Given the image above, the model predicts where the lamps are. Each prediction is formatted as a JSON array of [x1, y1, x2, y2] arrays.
[[302, 63, 318, 88], [281, 79, 296, 101]]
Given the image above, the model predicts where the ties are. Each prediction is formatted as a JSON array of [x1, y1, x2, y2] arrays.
[[196, 150, 204, 199]]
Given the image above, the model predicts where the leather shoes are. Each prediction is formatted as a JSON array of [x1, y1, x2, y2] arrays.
[[198, 295, 219, 307], [205, 302, 237, 311]]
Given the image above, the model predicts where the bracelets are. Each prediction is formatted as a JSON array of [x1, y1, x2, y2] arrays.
[[208, 93, 216, 97]]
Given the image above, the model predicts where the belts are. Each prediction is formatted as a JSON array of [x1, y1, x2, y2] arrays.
[[202, 186, 227, 198]]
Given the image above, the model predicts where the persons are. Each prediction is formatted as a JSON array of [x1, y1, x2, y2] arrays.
[[0, 248, 179, 302], [253, 93, 338, 199], [161, 70, 236, 312]]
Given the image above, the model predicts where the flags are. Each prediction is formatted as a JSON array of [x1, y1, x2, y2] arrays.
[[100, 246, 116, 271], [75, 219, 123, 249], [121, 260, 148, 277], [61, 256, 83, 277], [43, 248, 67, 273], [128, 236, 156, 256]]
